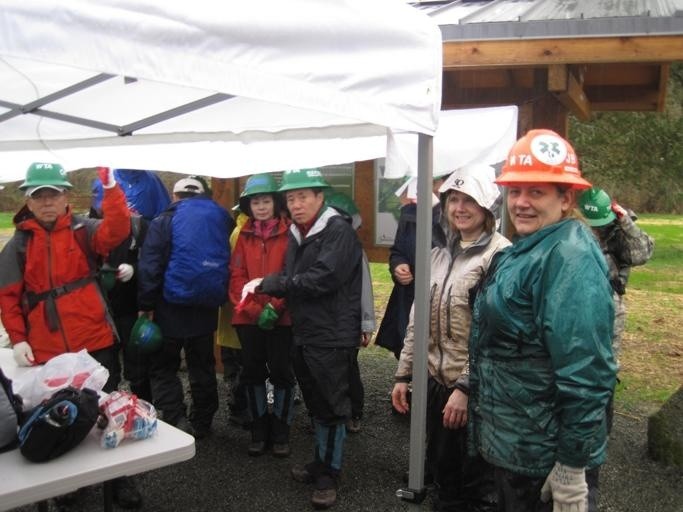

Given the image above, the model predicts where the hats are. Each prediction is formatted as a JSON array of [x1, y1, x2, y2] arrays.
[[173, 178, 205, 194], [24, 185, 66, 196]]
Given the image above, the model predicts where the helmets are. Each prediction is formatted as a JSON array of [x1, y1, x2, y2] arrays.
[[439, 163, 502, 219], [279, 168, 329, 191], [97, 267, 117, 294], [578, 190, 617, 227], [240, 174, 278, 197], [18, 163, 73, 190], [129, 312, 163, 352], [494, 129, 592, 189]]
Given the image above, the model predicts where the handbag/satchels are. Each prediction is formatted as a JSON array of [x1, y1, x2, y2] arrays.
[[18, 386, 99, 462]]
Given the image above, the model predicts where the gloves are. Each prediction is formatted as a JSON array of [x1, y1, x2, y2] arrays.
[[13, 340, 36, 366], [98, 167, 116, 189], [258, 304, 279, 330], [235, 278, 263, 313], [540, 463, 589, 512], [610, 200, 628, 221], [117, 264, 134, 282]]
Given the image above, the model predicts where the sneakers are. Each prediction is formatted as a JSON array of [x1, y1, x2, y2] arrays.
[[175, 390, 359, 505], [113, 476, 141, 507]]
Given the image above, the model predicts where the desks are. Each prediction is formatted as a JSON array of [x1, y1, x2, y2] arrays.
[[0, 350, 196, 512]]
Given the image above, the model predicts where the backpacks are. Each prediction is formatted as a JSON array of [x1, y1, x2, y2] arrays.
[[92, 169, 171, 219], [161, 198, 234, 307]]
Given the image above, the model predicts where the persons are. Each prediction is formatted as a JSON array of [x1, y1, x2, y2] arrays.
[[226, 174, 293, 458], [469, 129, 616, 512], [391, 161, 514, 511], [238, 167, 363, 509], [1, 161, 375, 441], [376, 170, 445, 411], [575, 186, 653, 436], [1, 161, 143, 509]]
